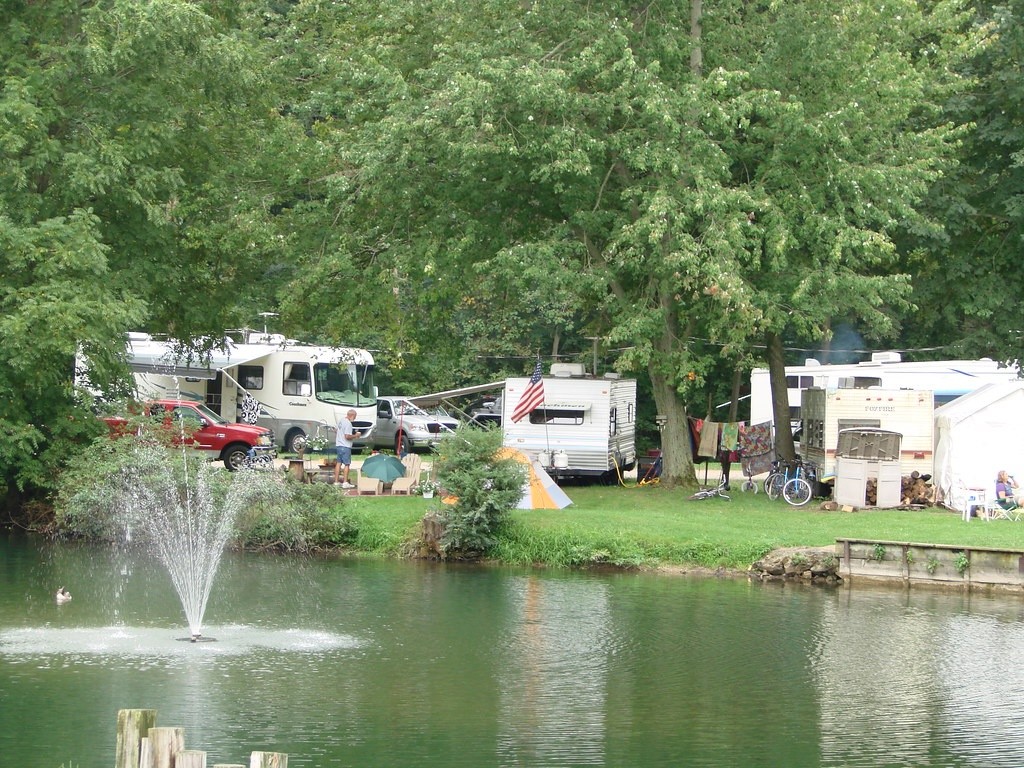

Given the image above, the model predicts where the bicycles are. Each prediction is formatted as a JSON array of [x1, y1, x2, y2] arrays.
[[689, 473, 731, 502], [740, 460, 758, 495], [763, 452, 812, 505]]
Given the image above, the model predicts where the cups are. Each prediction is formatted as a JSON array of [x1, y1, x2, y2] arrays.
[[1008, 475, 1012, 478], [356, 432, 361, 439]]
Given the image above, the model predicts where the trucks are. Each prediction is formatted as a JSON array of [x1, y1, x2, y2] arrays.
[[750, 351, 1024, 439], [71, 332, 379, 456]]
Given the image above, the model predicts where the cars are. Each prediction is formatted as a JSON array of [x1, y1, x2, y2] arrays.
[[376, 396, 462, 456], [468, 407, 502, 428]]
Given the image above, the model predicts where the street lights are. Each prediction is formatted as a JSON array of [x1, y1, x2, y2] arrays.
[[258, 312, 279, 334]]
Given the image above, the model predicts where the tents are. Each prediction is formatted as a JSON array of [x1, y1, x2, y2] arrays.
[[442, 447, 573, 508], [933, 380, 1024, 511]]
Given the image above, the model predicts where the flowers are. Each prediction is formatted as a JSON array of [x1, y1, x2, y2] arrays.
[[412, 480, 440, 494]]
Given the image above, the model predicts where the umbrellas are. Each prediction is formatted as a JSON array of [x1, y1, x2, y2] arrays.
[[362, 454, 405, 482]]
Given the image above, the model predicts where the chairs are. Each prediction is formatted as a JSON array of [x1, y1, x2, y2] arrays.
[[960, 479, 989, 522], [992, 485, 1021, 522]]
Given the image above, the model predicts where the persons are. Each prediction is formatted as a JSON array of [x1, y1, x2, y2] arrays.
[[996, 470, 1024, 509], [334, 410, 361, 488]]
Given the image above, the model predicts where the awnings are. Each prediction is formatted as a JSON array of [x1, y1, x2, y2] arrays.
[[129, 345, 275, 419], [398, 380, 506, 456]]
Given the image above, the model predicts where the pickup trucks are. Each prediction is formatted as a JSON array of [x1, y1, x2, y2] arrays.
[[98, 400, 278, 473]]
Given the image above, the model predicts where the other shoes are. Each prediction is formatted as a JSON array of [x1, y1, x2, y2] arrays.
[[334, 482, 343, 487], [342, 482, 355, 488]]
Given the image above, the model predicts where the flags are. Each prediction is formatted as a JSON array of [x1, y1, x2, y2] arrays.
[[512, 362, 544, 422]]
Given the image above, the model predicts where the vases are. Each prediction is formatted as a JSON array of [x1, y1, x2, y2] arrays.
[[423, 490, 434, 499]]
